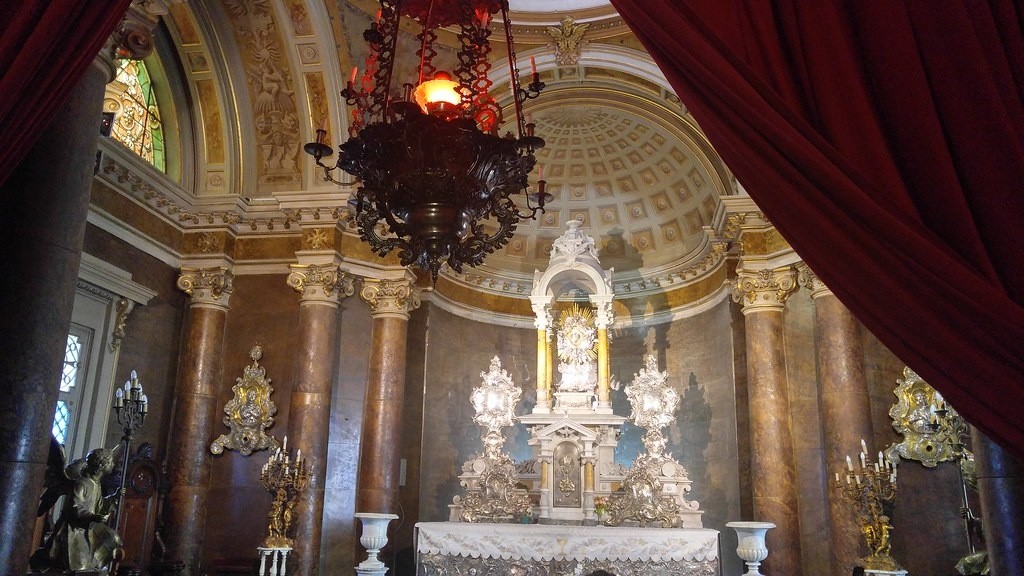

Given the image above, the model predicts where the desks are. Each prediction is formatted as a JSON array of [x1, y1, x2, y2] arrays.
[[412, 521, 733, 576]]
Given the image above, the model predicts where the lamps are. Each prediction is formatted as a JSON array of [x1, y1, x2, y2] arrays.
[[925, 390, 976, 555], [303, 0, 554, 290], [259, 435, 315, 549], [836, 439, 903, 571], [112, 369, 149, 532]]
